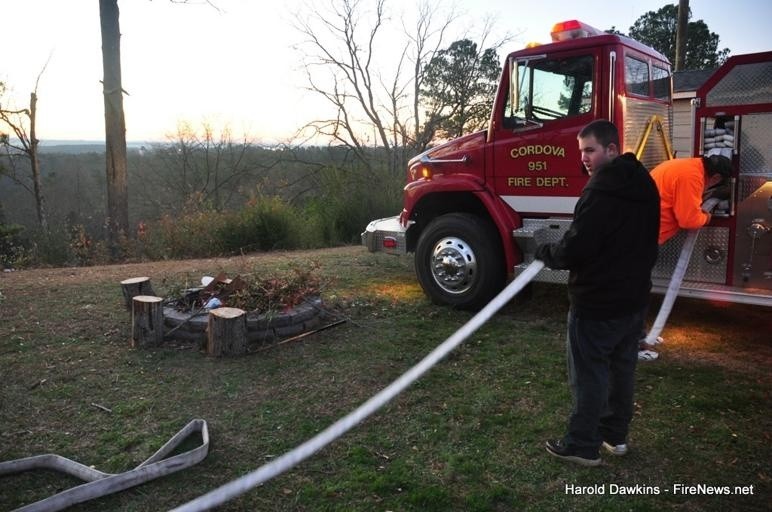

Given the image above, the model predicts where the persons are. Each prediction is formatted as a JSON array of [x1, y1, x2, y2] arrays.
[[636, 153, 733, 361], [534, 118, 661, 468]]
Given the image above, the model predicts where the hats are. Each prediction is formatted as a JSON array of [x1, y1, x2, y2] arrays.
[[711, 156, 733, 186]]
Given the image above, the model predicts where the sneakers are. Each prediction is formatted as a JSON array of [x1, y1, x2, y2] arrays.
[[546, 438, 602, 467], [643, 335, 664, 345], [598, 434, 628, 456], [637, 349, 659, 362]]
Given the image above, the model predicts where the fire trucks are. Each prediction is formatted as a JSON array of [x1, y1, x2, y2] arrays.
[[359, 21, 771, 309]]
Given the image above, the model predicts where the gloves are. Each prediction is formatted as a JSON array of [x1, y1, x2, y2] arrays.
[[534, 243, 552, 264], [702, 210, 713, 225]]
